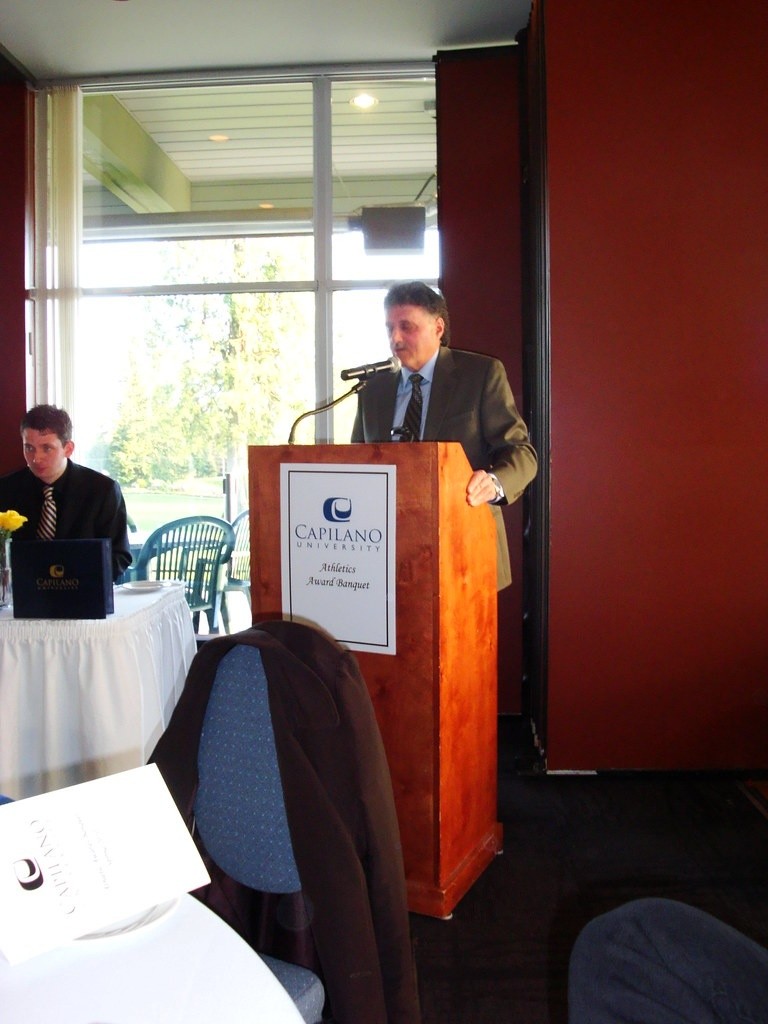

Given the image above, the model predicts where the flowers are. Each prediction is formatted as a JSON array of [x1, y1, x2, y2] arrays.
[[0, 510, 28, 602]]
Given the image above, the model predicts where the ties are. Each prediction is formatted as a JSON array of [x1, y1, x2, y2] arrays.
[[35, 486, 56, 540], [399, 374, 424, 442]]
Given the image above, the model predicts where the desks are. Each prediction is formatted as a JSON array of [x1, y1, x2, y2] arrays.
[[128, 540, 215, 581], [0, 580, 196, 801]]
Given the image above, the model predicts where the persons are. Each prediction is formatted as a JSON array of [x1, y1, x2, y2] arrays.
[[0, 404, 133, 582], [351, 282, 539, 594]]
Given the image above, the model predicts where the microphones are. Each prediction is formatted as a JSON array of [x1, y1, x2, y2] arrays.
[[342, 356, 402, 381]]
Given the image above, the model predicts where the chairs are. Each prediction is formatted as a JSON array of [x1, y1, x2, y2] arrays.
[[191, 510, 252, 635], [145, 620, 422, 1024], [121, 515, 236, 635]]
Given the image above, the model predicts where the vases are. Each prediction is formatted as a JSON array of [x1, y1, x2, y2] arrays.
[[0, 538, 12, 610]]
